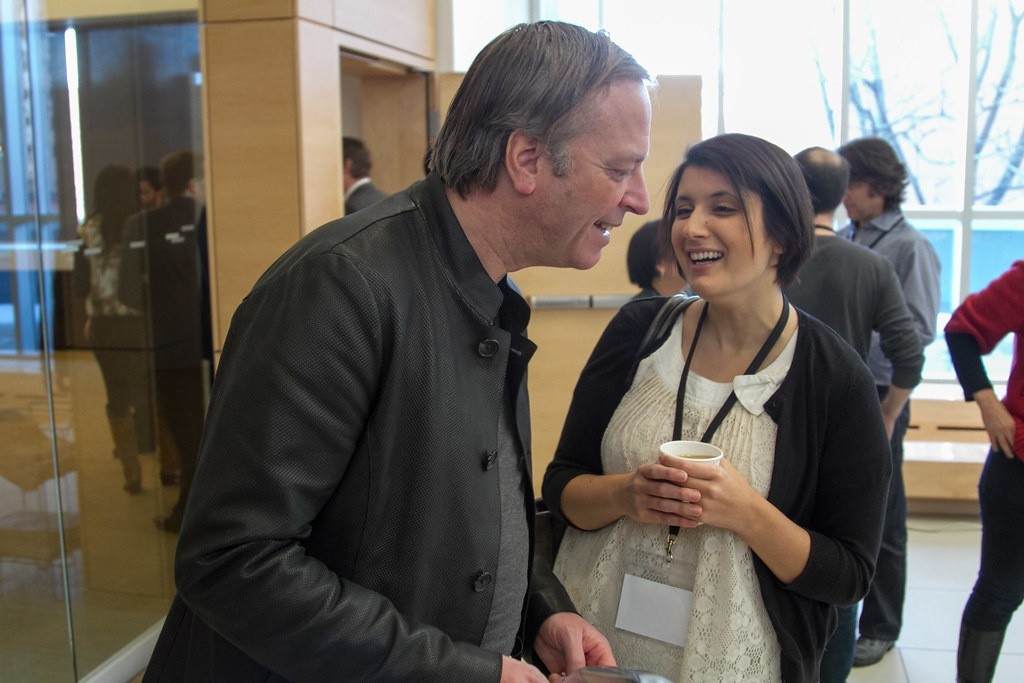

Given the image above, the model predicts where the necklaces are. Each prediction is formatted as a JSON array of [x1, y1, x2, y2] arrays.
[[850, 216, 904, 249], [815, 224, 836, 233]]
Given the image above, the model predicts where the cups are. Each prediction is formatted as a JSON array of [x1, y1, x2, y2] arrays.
[[659, 439, 723, 524]]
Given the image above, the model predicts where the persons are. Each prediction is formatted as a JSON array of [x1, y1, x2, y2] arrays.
[[140, 20, 653, 683], [628, 218, 689, 299], [835, 136, 942, 668], [784, 147, 925, 683], [944, 260, 1024, 683], [342, 137, 391, 215], [76, 152, 215, 533], [541, 133, 896, 683]]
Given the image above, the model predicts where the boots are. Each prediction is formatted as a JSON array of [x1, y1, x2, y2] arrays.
[[152, 408, 175, 485], [103, 403, 143, 494], [955, 622, 1005, 683]]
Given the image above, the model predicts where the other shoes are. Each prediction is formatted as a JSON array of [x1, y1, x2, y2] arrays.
[[852, 635, 896, 666], [154, 516, 174, 531]]
